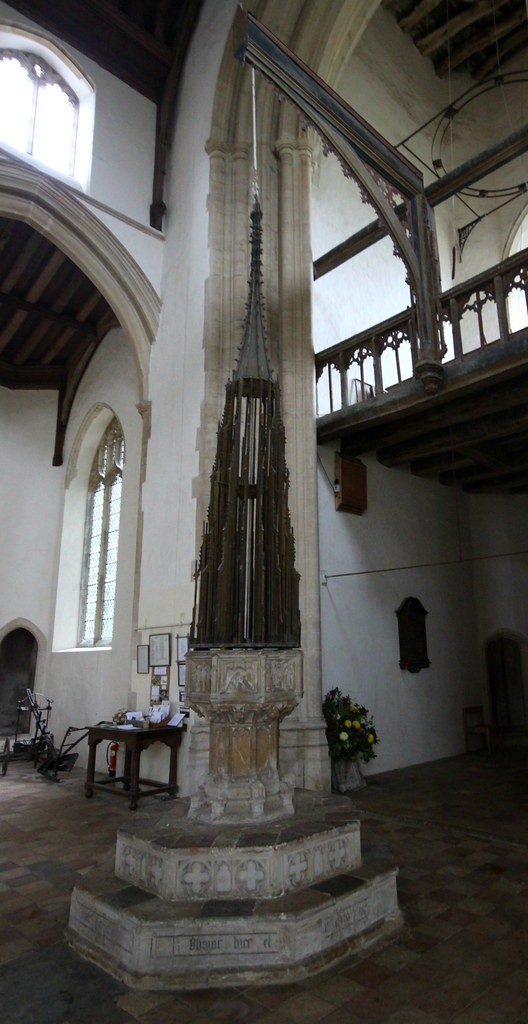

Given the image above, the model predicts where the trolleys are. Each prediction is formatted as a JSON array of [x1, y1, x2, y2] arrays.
[[0, 688, 113, 783]]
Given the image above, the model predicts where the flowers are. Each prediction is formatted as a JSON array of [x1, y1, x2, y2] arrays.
[[322, 687, 380, 764]]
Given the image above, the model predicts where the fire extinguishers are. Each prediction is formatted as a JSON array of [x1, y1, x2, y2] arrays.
[[106, 741, 119, 777]]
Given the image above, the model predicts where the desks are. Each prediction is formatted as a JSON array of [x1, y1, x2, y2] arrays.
[[84, 724, 188, 810]]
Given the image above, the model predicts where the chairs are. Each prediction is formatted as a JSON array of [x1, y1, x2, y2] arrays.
[[462, 706, 506, 756]]
